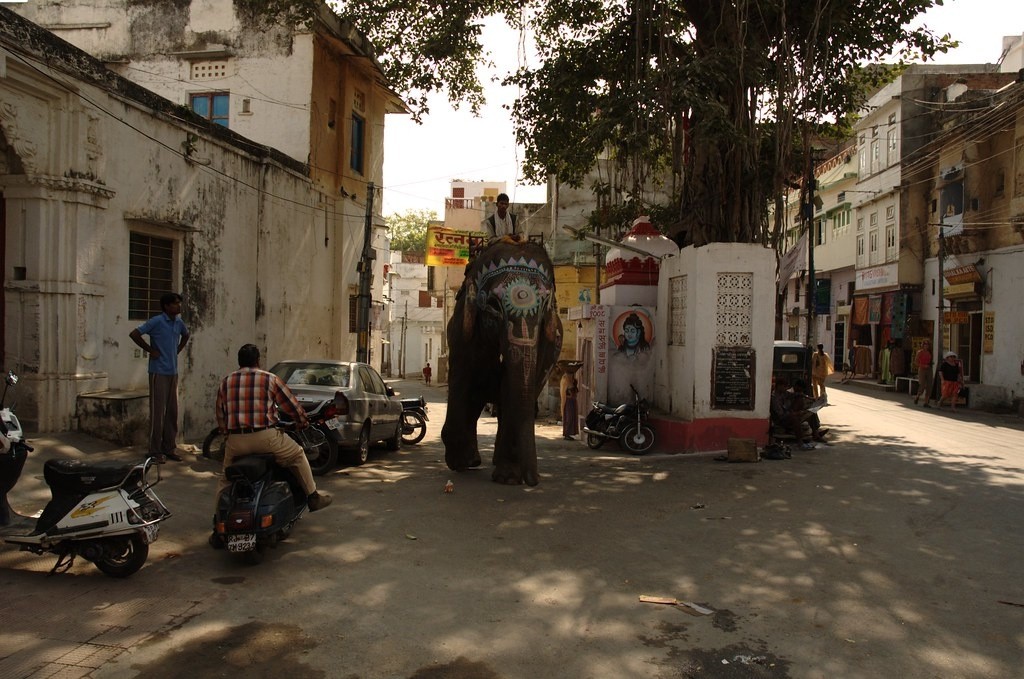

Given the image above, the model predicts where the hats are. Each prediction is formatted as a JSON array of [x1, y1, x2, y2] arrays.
[[943, 351, 958, 359]]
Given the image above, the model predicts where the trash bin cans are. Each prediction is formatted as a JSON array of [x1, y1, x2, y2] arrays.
[[771, 339, 813, 392]]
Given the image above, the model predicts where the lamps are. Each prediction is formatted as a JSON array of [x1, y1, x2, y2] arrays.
[[562, 224, 662, 262]]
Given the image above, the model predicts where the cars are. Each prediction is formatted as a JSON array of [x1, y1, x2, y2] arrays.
[[268, 359, 404, 465]]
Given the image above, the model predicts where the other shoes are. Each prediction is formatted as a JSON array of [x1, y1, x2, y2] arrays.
[[812, 428, 829, 441], [147, 450, 183, 463], [208, 533, 223, 550], [923, 403, 931, 408], [310, 495, 331, 512], [914, 399, 918, 404], [799, 443, 814, 450]]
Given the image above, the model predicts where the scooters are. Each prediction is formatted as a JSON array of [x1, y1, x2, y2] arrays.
[[0, 370, 172, 577], [208, 412, 310, 549]]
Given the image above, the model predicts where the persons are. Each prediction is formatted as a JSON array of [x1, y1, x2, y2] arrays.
[[812, 343, 834, 402], [485, 193, 526, 245], [423, 362, 432, 387], [914, 339, 934, 408], [208, 343, 333, 550], [559, 373, 579, 441], [939, 351, 963, 413], [128, 292, 190, 462]]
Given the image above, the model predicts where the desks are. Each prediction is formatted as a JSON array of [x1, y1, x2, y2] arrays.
[[894, 376, 920, 396]]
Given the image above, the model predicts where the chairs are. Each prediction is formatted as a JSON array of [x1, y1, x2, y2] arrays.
[[843, 346, 873, 380]]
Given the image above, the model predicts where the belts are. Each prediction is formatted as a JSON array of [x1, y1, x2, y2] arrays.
[[228, 424, 275, 435]]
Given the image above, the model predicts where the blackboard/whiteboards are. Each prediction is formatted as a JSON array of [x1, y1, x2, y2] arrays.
[[712, 349, 755, 411]]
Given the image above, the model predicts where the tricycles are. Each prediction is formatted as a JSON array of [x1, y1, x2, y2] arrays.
[[772, 340, 813, 393]]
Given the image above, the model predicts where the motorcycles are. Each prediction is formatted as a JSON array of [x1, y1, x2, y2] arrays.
[[397, 396, 429, 445], [203, 399, 336, 476], [582, 384, 656, 455]]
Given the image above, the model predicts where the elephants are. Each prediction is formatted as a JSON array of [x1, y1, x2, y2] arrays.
[[438, 233, 565, 486]]
